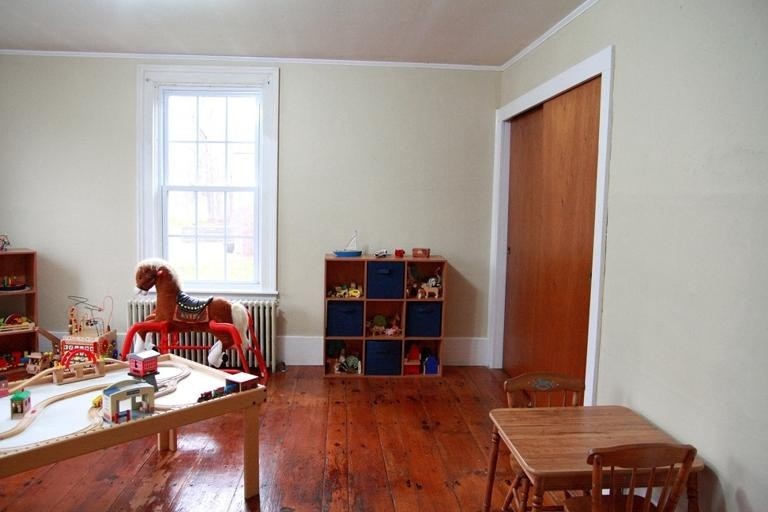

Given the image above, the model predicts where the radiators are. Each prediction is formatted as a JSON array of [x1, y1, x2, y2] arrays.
[[127, 297, 279, 373]]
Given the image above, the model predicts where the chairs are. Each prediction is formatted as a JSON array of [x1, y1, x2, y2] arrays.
[[501, 372, 585, 512], [563, 442, 697, 512]]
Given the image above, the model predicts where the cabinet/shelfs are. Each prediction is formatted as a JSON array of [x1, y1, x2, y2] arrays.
[[0, 248, 40, 382], [323, 253, 448, 379]]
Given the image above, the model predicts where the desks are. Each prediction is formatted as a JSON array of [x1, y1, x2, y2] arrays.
[[482, 405, 704, 512], [0, 353, 267, 512]]
